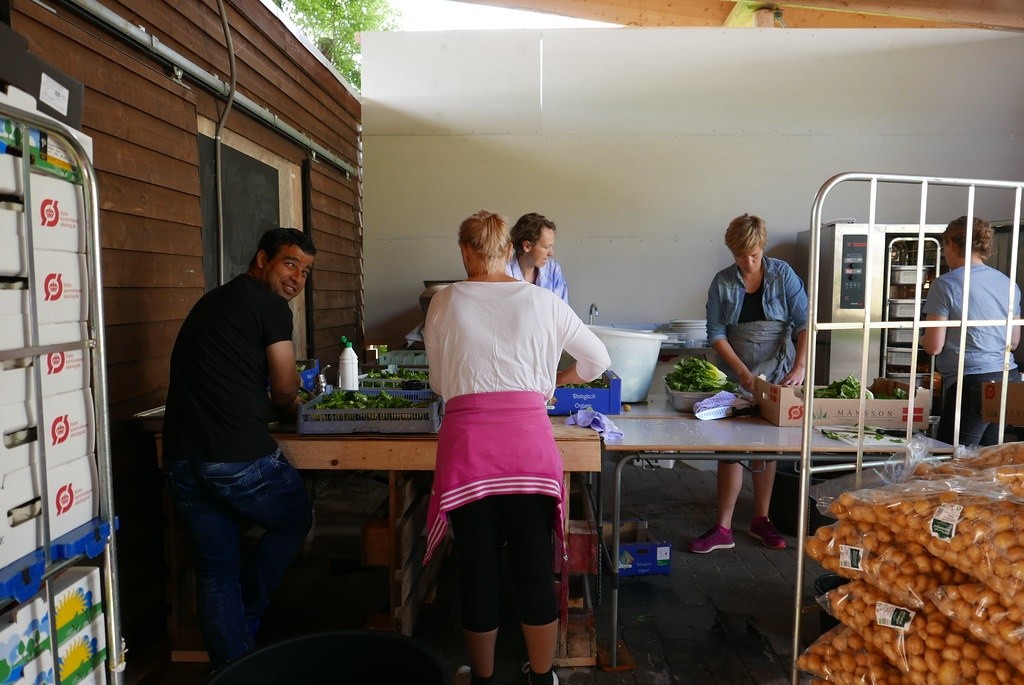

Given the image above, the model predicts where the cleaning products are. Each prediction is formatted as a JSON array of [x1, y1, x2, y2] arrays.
[[340, 335, 359, 389]]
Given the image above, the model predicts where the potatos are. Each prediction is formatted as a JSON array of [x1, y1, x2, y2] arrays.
[[795, 441, 1024, 685]]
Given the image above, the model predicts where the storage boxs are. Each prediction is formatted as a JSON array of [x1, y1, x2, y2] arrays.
[[300, 351, 443, 435], [751, 374, 930, 430], [0, 46, 122, 685], [602, 521, 674, 576], [544, 368, 621, 415], [982, 380, 1024, 426]]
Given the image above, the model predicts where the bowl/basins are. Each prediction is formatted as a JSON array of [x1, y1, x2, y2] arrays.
[[663, 319, 707, 346]]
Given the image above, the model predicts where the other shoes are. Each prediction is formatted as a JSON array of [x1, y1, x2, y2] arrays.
[[528, 670, 559, 685], [456, 666, 472, 685]]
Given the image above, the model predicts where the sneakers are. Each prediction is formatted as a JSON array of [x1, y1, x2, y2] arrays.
[[748, 520, 786, 548], [687, 526, 735, 553]]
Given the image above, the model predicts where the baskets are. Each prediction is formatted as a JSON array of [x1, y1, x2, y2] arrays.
[[295, 366, 443, 435]]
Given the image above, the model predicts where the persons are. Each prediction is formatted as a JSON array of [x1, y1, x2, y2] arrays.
[[918, 216, 1022, 462], [685, 214, 817, 553], [423, 210, 613, 685], [503, 213, 569, 305], [163, 228, 315, 671]]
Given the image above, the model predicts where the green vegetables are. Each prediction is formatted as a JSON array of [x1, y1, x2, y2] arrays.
[[664, 357, 739, 392], [815, 374, 911, 400], [295, 363, 439, 421], [560, 379, 609, 388]]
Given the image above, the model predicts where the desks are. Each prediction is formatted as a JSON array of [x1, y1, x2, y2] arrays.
[[132, 415, 602, 666], [600, 393, 955, 667]]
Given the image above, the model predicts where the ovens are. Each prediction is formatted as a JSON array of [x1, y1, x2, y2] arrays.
[[796, 220, 1023, 461]]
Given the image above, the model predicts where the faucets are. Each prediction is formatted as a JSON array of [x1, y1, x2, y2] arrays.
[[588, 302, 598, 325]]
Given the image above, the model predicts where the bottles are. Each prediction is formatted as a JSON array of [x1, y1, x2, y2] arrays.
[[338, 336, 360, 390], [419, 279, 464, 317]]
